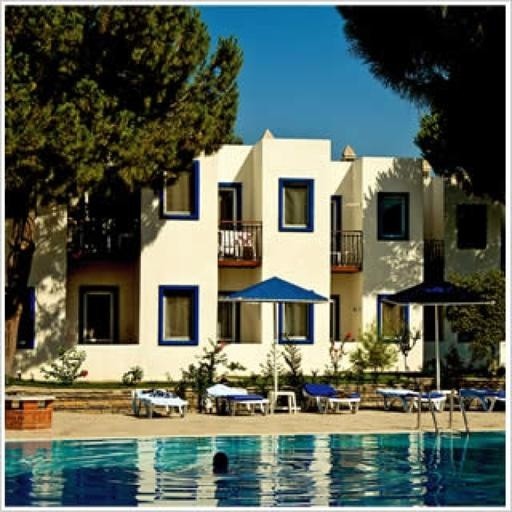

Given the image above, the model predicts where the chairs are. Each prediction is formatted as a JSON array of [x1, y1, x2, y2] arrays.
[[376, 389, 506, 412], [132, 388, 189, 419], [303, 384, 360, 414], [207, 383, 269, 416]]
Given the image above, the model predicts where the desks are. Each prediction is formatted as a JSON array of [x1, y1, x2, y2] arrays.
[[5, 395, 57, 430], [266, 391, 297, 416]]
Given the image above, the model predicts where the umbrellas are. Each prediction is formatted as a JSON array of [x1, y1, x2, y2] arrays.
[[381, 278, 495, 391], [217, 276, 332, 400]]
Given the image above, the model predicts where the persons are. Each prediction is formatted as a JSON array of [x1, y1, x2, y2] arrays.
[[212, 452, 229, 472]]
